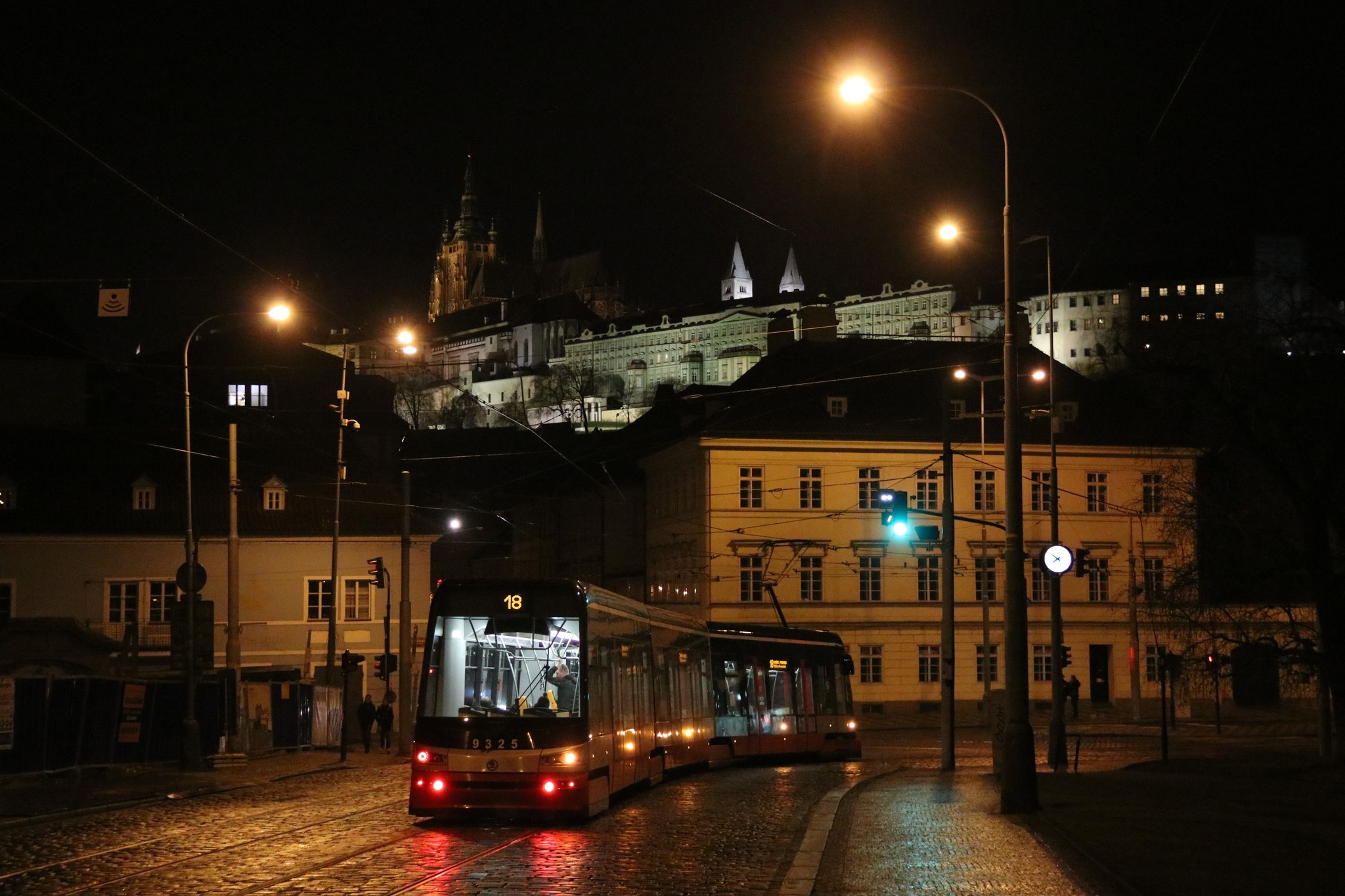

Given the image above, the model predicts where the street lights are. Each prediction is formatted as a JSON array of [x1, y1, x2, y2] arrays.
[[821, 68, 1042, 817], [327, 327, 415, 664], [948, 357, 1075, 772], [181, 290, 299, 767]]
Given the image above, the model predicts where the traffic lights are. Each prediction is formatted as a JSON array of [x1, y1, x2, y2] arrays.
[[1074, 548, 1092, 576], [368, 555, 399, 706], [876, 488, 908, 538]]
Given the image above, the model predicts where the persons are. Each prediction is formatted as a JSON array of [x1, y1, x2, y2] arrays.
[[375, 699, 394, 752], [357, 694, 376, 753], [1068, 675, 1081, 717], [1061, 675, 1070, 716], [546, 666, 581, 717]]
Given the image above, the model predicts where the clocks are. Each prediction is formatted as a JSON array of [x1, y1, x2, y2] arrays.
[[1043, 544, 1074, 574]]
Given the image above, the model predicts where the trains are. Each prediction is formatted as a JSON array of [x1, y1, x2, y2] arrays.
[[405, 570, 861, 829]]
